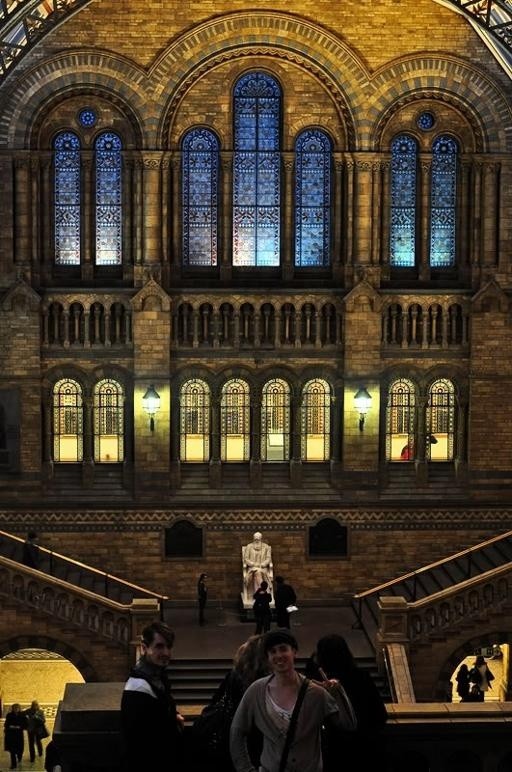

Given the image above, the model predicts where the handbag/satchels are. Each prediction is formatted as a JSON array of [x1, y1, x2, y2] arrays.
[[188, 702, 230, 754], [37, 725, 47, 740]]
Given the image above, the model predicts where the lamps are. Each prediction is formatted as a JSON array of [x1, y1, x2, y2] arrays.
[[354, 383, 372, 432], [143, 384, 160, 432]]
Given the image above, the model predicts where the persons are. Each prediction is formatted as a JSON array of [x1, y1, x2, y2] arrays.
[[242, 533, 275, 602], [401, 444, 414, 459], [22, 531, 42, 569], [123, 622, 387, 766], [253, 576, 297, 634], [4, 699, 49, 768], [455, 655, 495, 702], [197, 573, 208, 625]]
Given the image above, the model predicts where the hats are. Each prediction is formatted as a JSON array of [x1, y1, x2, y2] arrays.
[[265, 632, 298, 652]]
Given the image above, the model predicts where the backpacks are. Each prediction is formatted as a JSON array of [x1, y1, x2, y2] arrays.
[[255, 594, 269, 613], [281, 582, 296, 605]]
